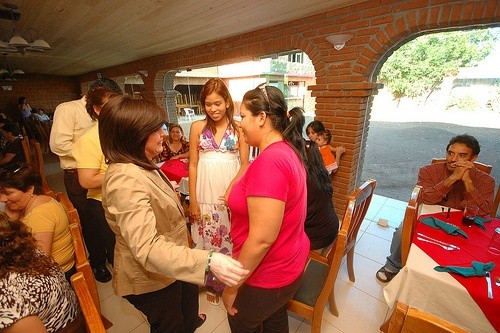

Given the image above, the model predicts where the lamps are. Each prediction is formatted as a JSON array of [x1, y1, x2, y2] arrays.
[[0, 3, 52, 91], [325, 33, 353, 51]]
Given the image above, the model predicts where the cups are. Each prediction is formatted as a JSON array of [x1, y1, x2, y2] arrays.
[[462, 204, 479, 227], [487, 227, 500, 256]]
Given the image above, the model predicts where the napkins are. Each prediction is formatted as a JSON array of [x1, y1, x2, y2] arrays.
[[421, 214, 467, 239], [474, 215, 491, 230], [433, 261, 494, 277]]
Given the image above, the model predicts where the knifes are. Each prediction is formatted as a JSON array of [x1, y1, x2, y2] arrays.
[[485, 271, 493, 299], [446, 207, 451, 221], [417, 232, 460, 249]]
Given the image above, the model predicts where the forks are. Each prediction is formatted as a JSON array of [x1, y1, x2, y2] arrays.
[[417, 237, 454, 250]]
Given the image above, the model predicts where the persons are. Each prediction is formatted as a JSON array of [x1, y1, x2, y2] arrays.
[[377, 133, 496, 282], [0, 77, 346, 333]]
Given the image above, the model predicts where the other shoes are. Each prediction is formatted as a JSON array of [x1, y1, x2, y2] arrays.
[[196, 313, 207, 328], [89, 260, 112, 283]]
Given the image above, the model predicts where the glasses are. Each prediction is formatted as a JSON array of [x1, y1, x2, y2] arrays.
[[0, 162, 23, 174], [256, 81, 272, 112]]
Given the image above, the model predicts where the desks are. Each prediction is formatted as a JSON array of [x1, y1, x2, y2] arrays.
[[381, 204, 500, 333], [155, 158, 190, 200]]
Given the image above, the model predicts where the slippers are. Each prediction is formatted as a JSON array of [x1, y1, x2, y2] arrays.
[[207, 289, 221, 306], [376, 266, 394, 283]]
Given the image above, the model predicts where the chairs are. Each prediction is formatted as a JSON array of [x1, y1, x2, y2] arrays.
[[401, 158, 500, 265], [0, 107, 378, 332]]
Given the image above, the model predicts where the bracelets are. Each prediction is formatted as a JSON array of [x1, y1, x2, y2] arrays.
[[205, 249, 214, 272]]
